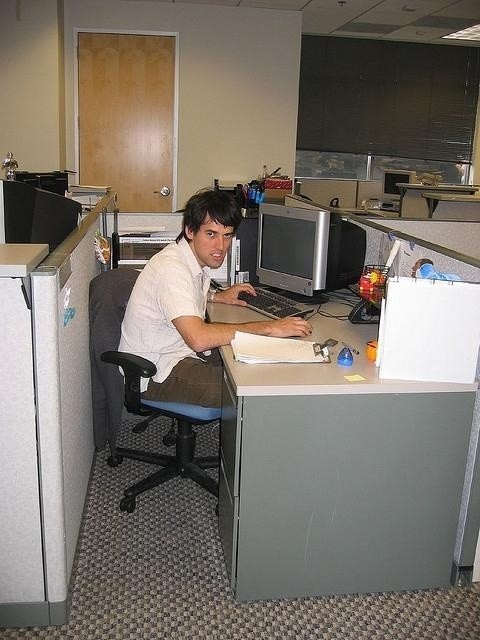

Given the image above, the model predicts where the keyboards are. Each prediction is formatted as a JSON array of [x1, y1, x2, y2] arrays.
[[239, 288, 313, 321]]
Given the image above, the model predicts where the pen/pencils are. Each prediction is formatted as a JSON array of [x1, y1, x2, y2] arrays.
[[236, 180, 265, 209]]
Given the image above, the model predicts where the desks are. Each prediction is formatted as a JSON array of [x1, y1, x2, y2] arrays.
[[205, 282, 479, 604], [337, 204, 400, 219]]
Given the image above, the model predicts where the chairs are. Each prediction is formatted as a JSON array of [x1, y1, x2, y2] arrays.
[[86, 269, 223, 513]]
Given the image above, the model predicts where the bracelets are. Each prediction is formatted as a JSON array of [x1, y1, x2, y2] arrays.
[[209, 294, 215, 303]]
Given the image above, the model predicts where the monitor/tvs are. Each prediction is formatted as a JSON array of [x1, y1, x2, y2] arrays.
[[258, 202, 366, 303], [382, 170, 417, 203]]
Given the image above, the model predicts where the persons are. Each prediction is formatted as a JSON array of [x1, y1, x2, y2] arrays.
[[114, 184, 313, 410]]
